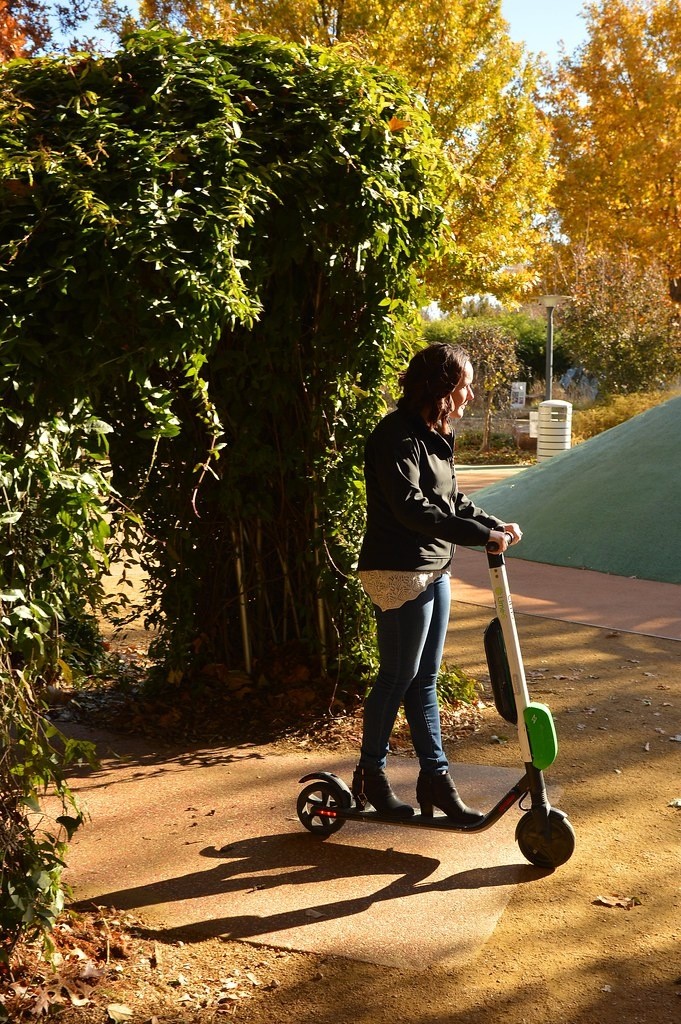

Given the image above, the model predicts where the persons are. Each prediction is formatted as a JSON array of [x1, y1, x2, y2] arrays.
[[349, 343, 523, 825]]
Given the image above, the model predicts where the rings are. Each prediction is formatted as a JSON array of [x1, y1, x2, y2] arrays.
[[520, 532, 523, 535]]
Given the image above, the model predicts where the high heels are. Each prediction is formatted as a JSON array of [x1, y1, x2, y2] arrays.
[[416, 769, 484, 824], [351, 764, 414, 819]]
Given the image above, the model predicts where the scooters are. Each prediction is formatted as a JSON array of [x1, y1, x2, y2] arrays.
[[296, 525, 575, 869]]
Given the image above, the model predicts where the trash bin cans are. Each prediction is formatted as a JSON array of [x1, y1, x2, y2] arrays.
[[535, 400, 573, 462]]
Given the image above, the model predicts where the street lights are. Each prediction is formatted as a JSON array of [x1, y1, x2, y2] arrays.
[[532, 294, 572, 399]]
